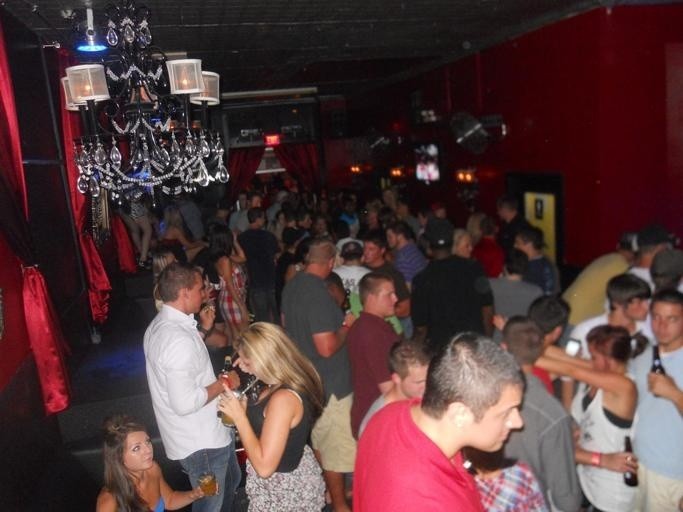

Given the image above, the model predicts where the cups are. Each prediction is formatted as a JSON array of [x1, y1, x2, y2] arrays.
[[222, 392, 241, 426], [312, 332, 338, 358], [197, 472, 217, 498]]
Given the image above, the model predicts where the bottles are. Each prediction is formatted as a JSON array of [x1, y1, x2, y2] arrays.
[[218, 355, 236, 415], [624, 435, 639, 487], [649, 344, 665, 398]]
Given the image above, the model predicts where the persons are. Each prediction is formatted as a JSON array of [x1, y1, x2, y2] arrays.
[[92, 409, 221, 512], [114, 168, 681, 510]]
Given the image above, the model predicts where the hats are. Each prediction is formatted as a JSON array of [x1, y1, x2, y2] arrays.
[[636, 226, 674, 246], [649, 249, 682, 274], [425, 222, 452, 246], [342, 242, 361, 256]]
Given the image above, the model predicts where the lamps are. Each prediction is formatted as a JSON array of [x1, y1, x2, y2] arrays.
[[48, 0, 231, 206]]
